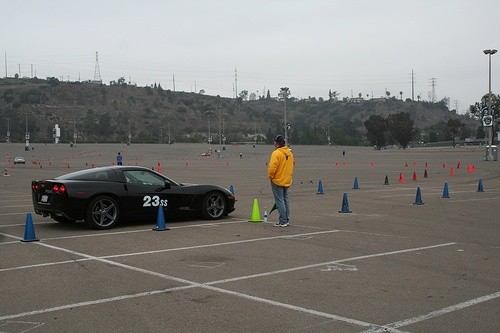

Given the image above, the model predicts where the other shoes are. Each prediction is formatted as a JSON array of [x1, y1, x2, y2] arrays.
[[274, 222, 289, 227]]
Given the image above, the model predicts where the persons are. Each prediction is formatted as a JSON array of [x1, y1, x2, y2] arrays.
[[116, 152, 123, 165], [268, 135, 295, 227]]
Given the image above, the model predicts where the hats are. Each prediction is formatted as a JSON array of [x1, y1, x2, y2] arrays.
[[272, 135, 284, 142]]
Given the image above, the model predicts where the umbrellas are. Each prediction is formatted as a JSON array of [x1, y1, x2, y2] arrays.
[[269, 203, 277, 214]]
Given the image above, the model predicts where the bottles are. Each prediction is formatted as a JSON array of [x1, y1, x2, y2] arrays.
[[263, 210, 268, 222]]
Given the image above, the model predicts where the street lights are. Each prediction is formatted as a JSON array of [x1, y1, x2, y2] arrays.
[[482, 50, 497, 160]]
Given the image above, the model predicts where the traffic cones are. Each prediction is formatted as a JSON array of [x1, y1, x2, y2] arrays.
[[152, 205, 169, 231], [337, 192, 352, 213], [336, 160, 475, 178], [20, 212, 39, 242], [33, 158, 268, 172], [441, 181, 450, 198], [383, 176, 390, 185], [413, 171, 416, 181], [413, 186, 425, 204], [399, 172, 404, 182], [316, 180, 325, 194], [352, 177, 360, 189], [476, 179, 485, 192], [230, 184, 237, 201], [248, 199, 263, 222]]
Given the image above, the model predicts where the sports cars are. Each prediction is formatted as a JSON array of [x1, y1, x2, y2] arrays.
[[14, 157, 25, 164], [202, 153, 210, 156], [31, 165, 236, 229]]
